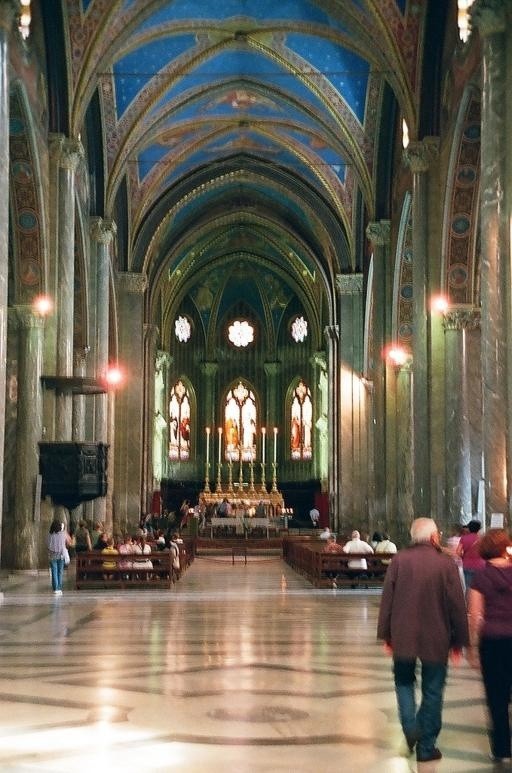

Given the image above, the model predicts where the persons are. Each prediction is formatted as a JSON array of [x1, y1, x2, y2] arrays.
[[454, 519, 481, 602], [319, 526, 398, 589], [459, 525, 512, 764], [73, 520, 91, 579], [93, 524, 184, 582], [46, 518, 68, 593], [376, 515, 467, 765], [364, 531, 391, 577]]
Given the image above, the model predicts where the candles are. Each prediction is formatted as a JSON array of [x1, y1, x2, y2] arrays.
[[205, 425, 277, 464]]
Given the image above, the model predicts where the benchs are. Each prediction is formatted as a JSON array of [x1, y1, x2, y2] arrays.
[[281, 535, 402, 590], [76, 534, 203, 588]]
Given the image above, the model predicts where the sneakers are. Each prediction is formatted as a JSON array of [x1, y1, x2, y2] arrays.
[[487, 752, 512, 765], [52, 589, 63, 594], [407, 739, 443, 762]]
